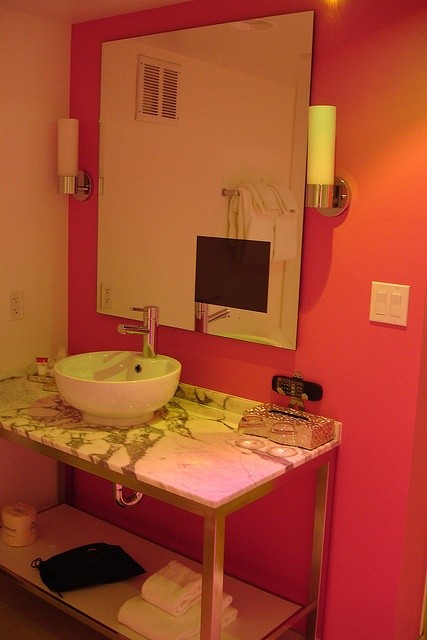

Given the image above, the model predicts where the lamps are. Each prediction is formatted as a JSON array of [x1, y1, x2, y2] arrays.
[[307, 106, 352, 218], [56, 117, 92, 202]]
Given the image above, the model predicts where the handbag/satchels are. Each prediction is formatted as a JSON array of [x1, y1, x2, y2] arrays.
[[31, 543, 146, 598]]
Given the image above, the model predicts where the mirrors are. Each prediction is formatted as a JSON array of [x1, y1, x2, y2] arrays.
[[95, 10, 314, 352]]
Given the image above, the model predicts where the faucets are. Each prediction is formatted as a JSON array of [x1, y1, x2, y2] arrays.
[[194, 301, 230, 334], [118, 306, 160, 359]]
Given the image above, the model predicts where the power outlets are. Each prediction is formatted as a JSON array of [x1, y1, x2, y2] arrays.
[[101, 283, 113, 308], [9, 294, 23, 320]]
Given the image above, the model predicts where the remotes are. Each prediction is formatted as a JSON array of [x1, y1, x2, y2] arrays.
[[271, 376, 323, 401]]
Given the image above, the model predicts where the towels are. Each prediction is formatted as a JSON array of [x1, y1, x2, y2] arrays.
[[270, 182, 300, 263], [228, 181, 283, 242], [118, 591, 238, 640], [141, 559, 202, 617]]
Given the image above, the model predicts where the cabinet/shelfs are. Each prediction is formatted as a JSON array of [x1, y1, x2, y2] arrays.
[[1, 369, 343, 640]]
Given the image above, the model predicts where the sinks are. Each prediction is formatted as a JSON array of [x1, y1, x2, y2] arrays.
[[216, 334, 281, 350], [54, 351, 182, 424]]
